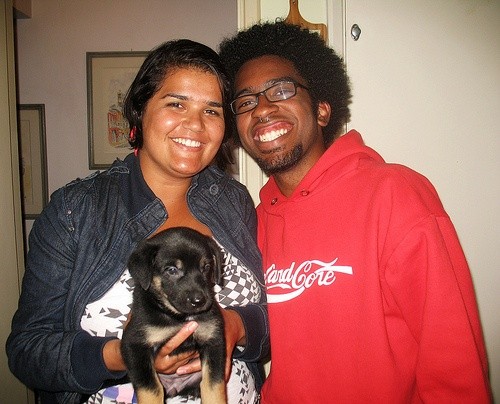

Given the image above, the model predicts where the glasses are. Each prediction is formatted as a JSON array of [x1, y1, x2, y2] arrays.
[[229, 77, 312, 117]]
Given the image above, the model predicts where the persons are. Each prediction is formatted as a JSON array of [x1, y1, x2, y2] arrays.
[[212, 19, 493, 404], [4, 39, 272, 404]]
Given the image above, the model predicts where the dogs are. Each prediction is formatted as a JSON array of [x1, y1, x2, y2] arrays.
[[120, 226, 228, 404]]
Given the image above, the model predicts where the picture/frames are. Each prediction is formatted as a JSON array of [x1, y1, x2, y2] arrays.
[[86, 51, 151, 170], [19, 104, 49, 220]]
